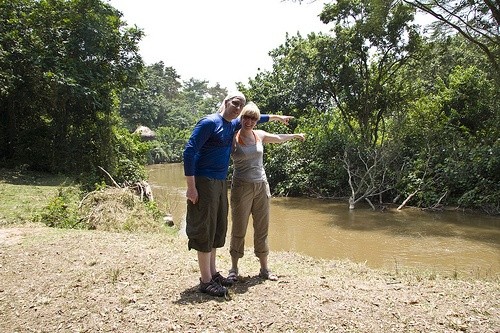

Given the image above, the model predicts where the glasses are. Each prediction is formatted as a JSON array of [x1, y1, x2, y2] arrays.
[[242, 114, 258, 122], [232, 100, 245, 110]]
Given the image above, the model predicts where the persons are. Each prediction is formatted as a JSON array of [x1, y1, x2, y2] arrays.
[[227, 101, 305, 284], [183, 89, 294, 297]]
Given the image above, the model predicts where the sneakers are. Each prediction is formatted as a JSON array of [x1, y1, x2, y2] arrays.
[[212, 271, 234, 287], [199, 277, 228, 297]]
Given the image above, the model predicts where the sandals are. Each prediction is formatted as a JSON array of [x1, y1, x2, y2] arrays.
[[259, 268, 279, 282], [227, 267, 238, 282]]
